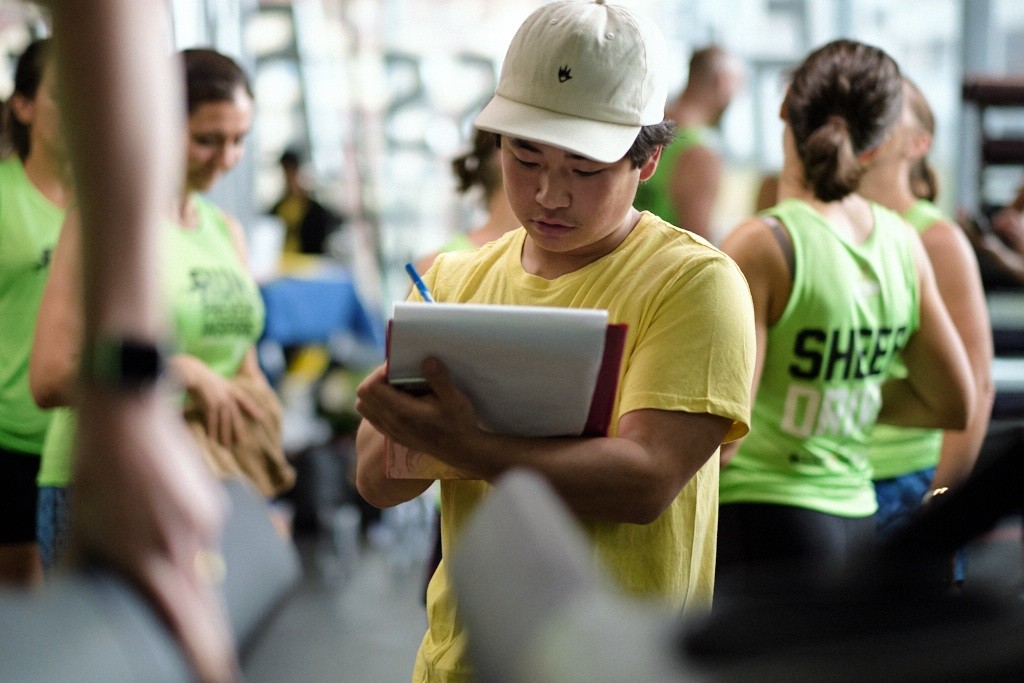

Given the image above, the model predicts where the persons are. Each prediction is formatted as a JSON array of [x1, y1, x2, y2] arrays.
[[713, 39, 980, 614], [0, 0, 1024, 683]]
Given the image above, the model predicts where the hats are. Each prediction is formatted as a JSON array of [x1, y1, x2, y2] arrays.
[[473, 1, 669, 163]]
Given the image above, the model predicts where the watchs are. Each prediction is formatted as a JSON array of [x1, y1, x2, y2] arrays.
[[76, 334, 173, 391]]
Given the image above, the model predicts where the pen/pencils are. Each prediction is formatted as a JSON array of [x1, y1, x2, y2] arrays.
[[404, 263, 435, 303]]
